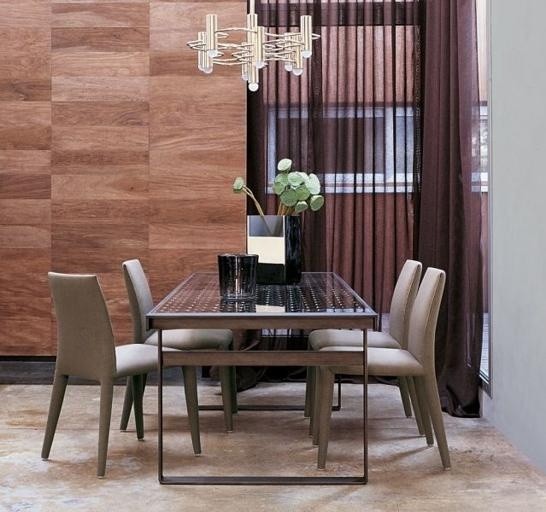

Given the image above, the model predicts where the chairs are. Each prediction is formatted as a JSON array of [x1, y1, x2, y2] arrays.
[[119, 258, 239, 434], [317, 266, 451, 469], [305, 258, 423, 438], [41, 271, 202, 476]]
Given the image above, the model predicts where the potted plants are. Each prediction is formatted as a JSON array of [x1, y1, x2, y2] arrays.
[[232, 157, 324, 286]]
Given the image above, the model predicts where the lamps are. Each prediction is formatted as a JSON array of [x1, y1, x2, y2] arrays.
[[186, 0, 322, 92]]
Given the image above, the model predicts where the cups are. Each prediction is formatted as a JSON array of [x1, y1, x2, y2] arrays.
[[218, 253, 259, 300]]
